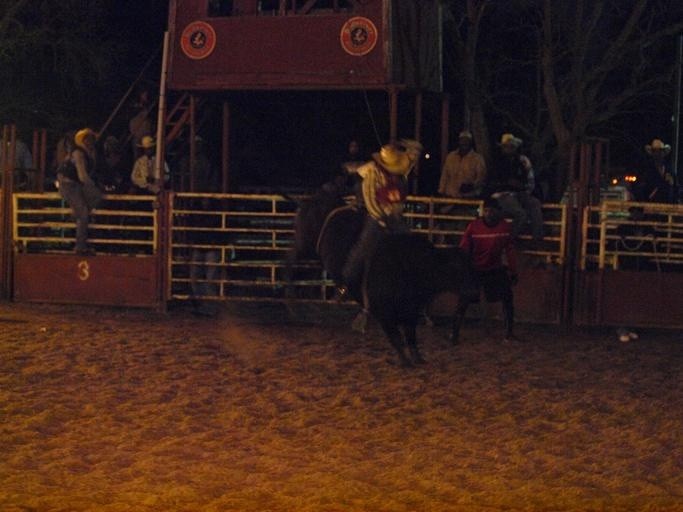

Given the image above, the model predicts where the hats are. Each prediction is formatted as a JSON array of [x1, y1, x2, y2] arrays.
[[497, 134, 522, 148], [646, 140, 671, 156], [75, 128, 100, 146], [459, 131, 473, 139], [371, 145, 410, 174], [136, 137, 156, 148]]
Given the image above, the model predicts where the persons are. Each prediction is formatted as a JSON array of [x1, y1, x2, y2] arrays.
[[533, 144, 579, 237], [7, 128, 32, 237], [58, 128, 219, 308], [335, 132, 544, 342], [630, 139, 674, 205], [608, 207, 657, 269]]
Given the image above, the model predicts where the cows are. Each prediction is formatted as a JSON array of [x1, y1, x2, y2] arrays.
[[279, 177, 483, 370]]
